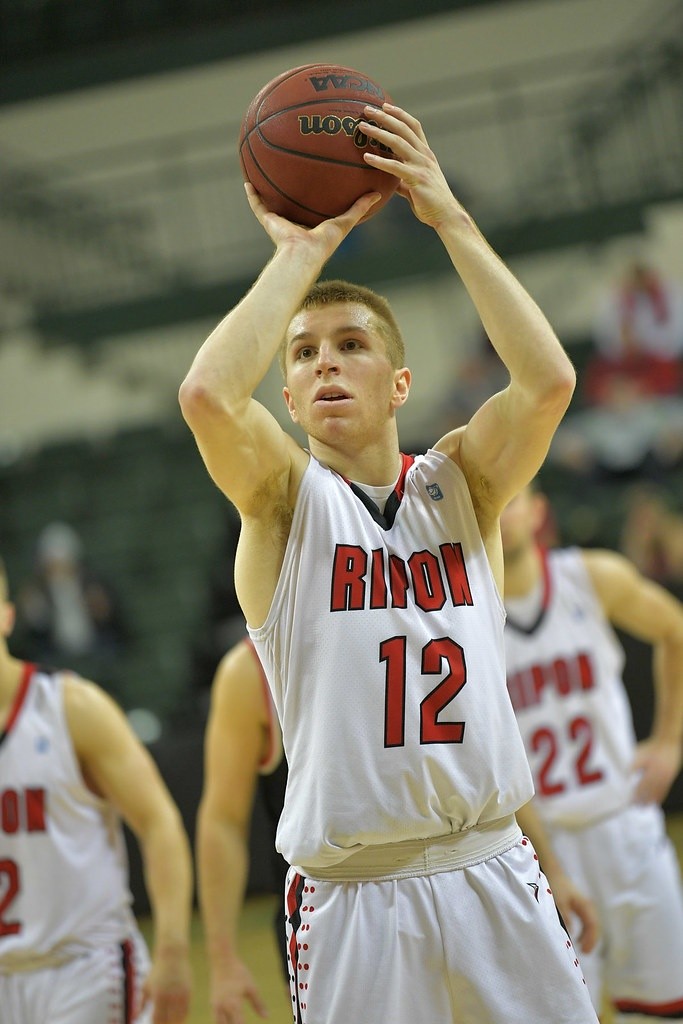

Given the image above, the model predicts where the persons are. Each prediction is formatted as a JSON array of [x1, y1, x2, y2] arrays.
[[0, 455, 683, 1024], [177, 101, 601, 1023]]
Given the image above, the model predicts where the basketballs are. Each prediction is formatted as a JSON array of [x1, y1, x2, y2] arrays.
[[243, 62, 399, 221]]
